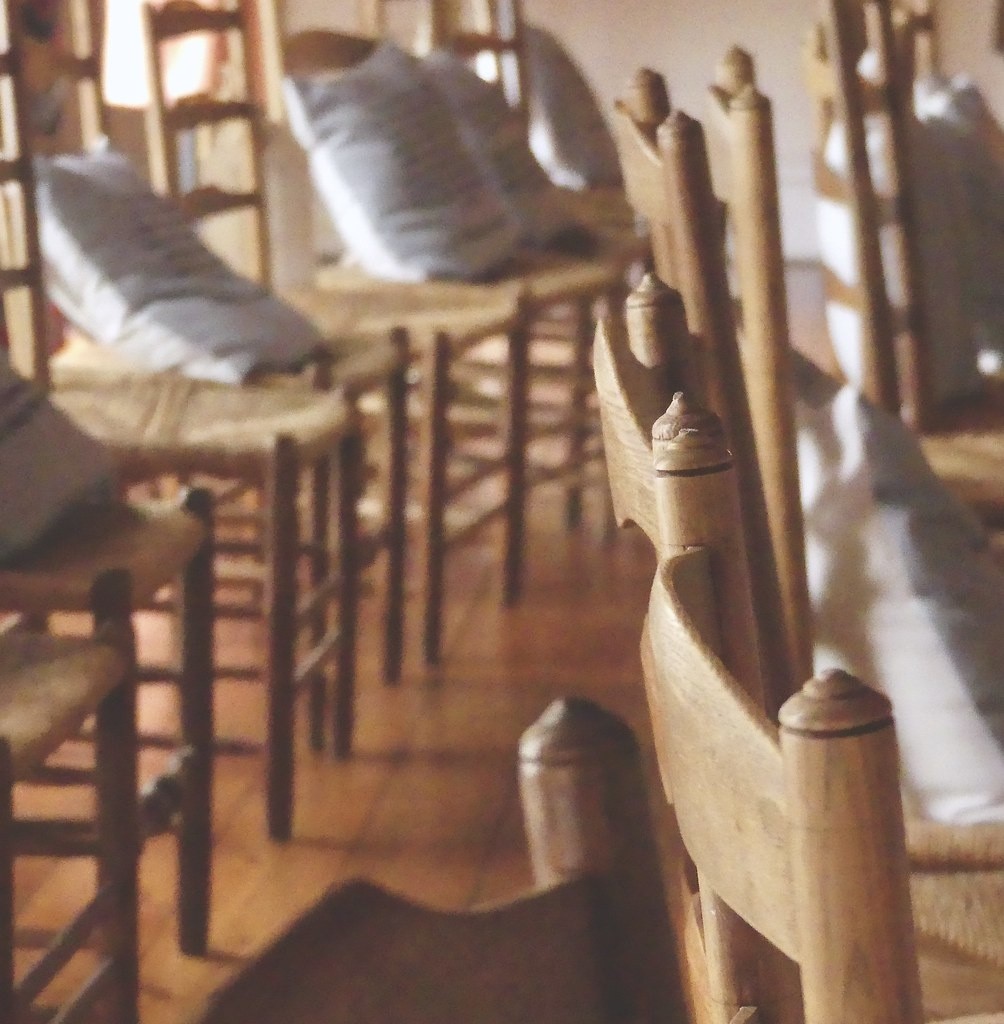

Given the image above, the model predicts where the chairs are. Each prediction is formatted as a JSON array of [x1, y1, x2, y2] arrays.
[[0, 0, 1003, 1024]]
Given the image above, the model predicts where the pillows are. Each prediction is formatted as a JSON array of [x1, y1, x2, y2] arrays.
[[794, 383, 1003, 824], [812, 41, 1004, 406], [0, 349, 126, 569], [282, 46, 517, 284], [514, 23, 624, 193], [917, 66, 1004, 208], [36, 151, 324, 387], [413, 47, 555, 236]]
